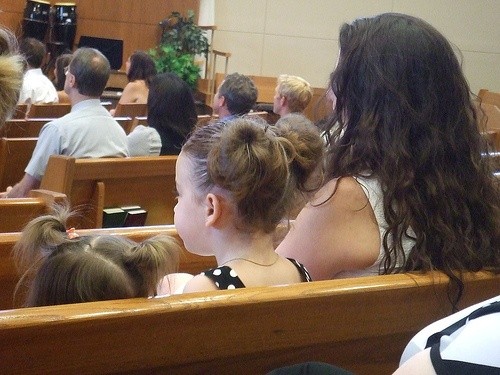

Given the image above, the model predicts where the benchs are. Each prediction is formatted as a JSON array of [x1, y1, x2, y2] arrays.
[[0, 90, 500, 375]]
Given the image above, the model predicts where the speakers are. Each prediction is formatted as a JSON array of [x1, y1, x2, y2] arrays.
[[78, 35, 124, 70]]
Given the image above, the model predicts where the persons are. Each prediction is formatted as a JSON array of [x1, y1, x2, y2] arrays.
[[0, 30, 324, 308], [265, 296, 500, 375], [275, 12, 500, 314]]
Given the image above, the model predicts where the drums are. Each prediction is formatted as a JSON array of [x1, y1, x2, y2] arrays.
[[21, 18, 49, 44], [22, 0, 51, 22], [50, 23, 77, 53], [52, 3, 77, 25]]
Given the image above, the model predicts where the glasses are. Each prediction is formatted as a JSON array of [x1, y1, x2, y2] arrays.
[[64, 67, 69, 76]]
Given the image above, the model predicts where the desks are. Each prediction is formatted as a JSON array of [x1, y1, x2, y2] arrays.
[[106, 69, 128, 89]]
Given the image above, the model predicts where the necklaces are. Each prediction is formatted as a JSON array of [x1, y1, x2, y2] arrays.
[[218, 257, 280, 266]]
